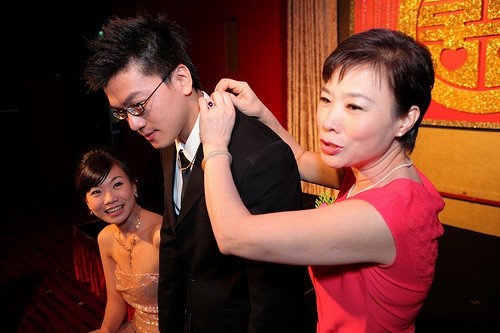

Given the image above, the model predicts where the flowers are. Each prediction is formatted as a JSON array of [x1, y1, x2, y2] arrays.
[[314, 192, 337, 210]]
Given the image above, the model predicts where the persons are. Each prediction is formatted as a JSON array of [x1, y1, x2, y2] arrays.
[[75, 150, 164, 333], [85, 15, 302, 333], [198, 28, 445, 333]]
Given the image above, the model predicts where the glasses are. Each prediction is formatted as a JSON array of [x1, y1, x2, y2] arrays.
[[110, 70, 170, 121]]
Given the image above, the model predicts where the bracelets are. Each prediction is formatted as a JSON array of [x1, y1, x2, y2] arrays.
[[201, 150, 232, 170]]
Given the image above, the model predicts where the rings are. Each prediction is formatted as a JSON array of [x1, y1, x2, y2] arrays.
[[207, 101, 215, 108]]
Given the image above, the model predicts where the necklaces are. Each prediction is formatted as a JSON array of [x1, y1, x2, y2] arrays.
[[177, 152, 196, 170], [346, 155, 413, 199], [115, 205, 141, 268]]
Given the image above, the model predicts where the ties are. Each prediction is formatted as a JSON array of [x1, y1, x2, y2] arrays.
[[180, 150, 190, 217]]
